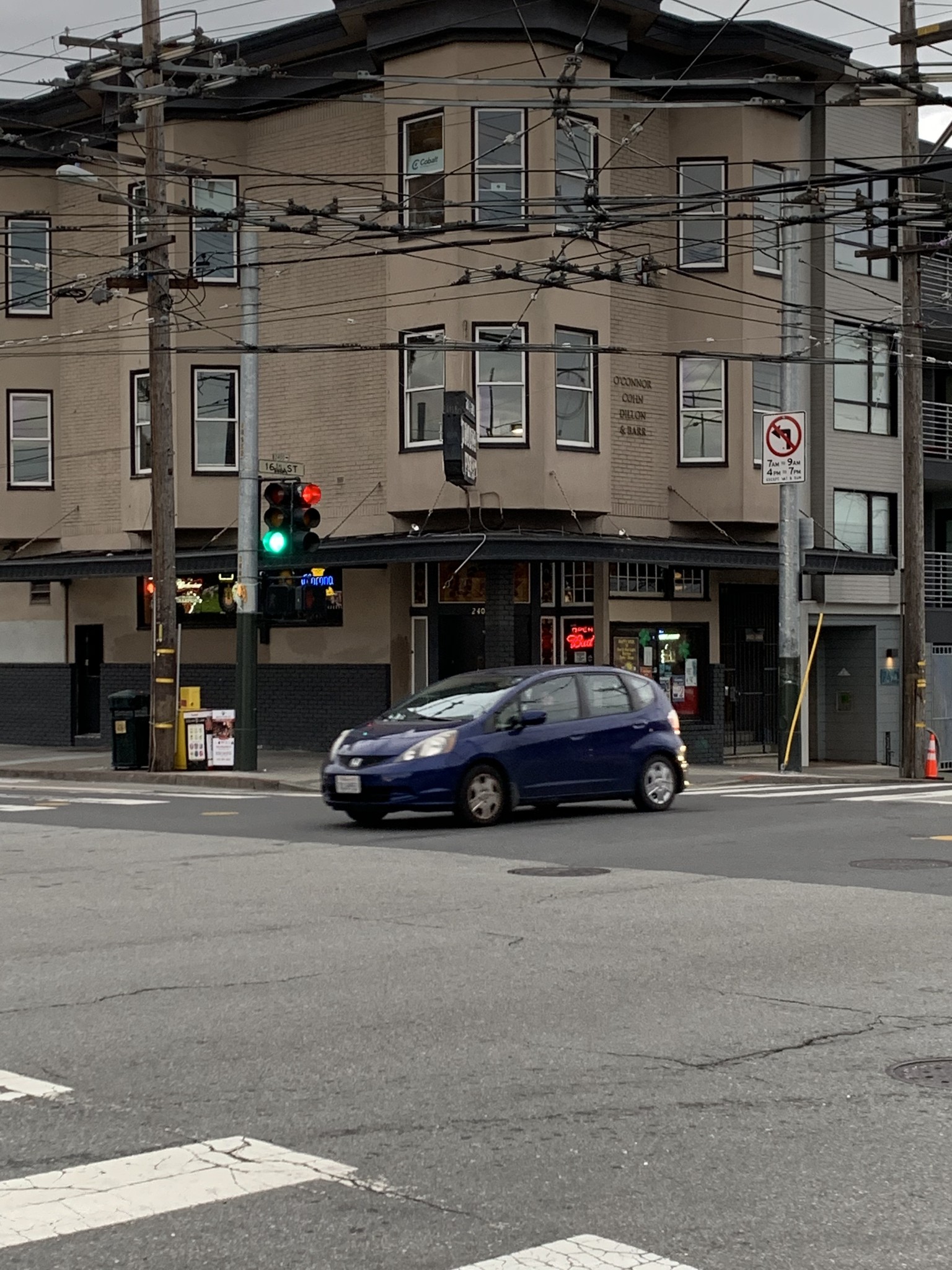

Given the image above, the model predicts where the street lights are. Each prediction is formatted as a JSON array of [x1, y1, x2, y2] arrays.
[[54, 162, 180, 773]]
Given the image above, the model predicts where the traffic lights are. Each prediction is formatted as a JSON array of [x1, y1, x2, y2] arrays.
[[291, 481, 320, 558], [263, 483, 293, 556]]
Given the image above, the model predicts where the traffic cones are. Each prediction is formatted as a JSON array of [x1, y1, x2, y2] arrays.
[[922, 733, 945, 780]]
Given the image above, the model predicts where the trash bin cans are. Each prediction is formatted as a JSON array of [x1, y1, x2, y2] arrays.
[[184, 711, 235, 769], [106, 689, 150, 770], [174, 687, 201, 770]]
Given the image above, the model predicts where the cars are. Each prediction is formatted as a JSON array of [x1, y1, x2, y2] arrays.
[[320, 664, 691, 825]]
